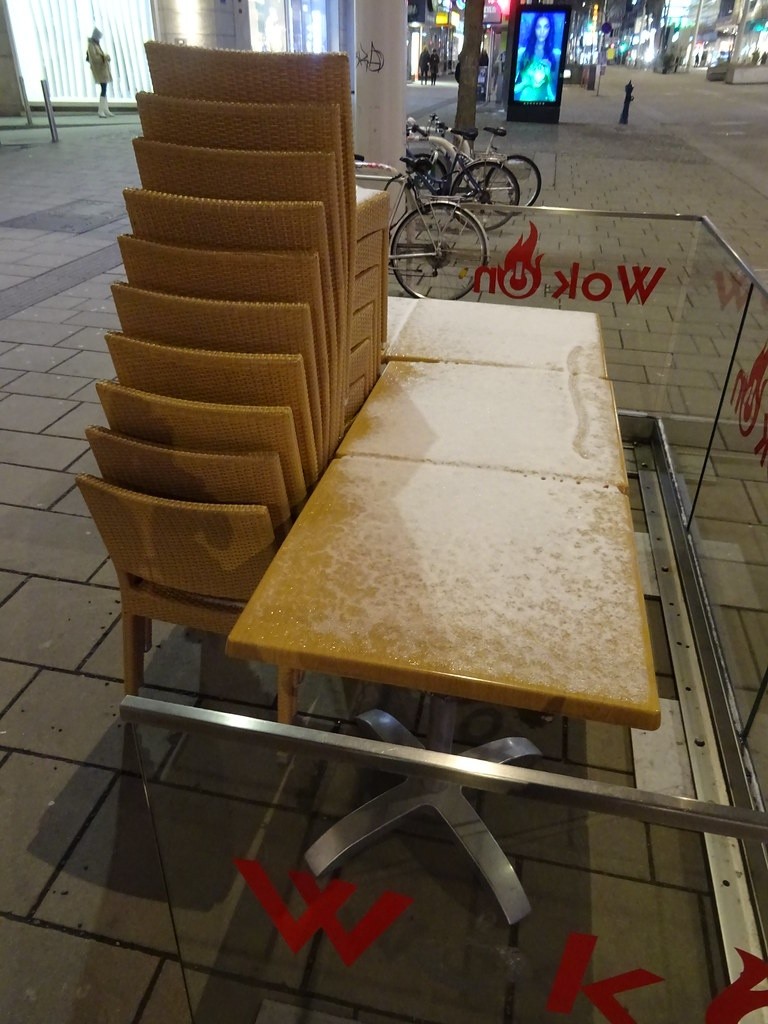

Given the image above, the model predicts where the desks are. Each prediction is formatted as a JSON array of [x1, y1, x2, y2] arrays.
[[221, 291, 658, 927]]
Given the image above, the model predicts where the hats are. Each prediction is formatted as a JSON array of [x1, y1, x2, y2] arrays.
[[91, 26, 103, 39]]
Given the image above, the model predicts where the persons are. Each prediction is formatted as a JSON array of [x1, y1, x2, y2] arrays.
[[694, 52, 700, 68], [88, 26, 115, 118], [480, 49, 488, 66], [419, 45, 440, 86], [674, 56, 679, 72], [752, 50, 767, 65], [513, 12, 556, 102]]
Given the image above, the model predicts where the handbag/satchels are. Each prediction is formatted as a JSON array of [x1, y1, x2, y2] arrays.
[[86, 50, 90, 62]]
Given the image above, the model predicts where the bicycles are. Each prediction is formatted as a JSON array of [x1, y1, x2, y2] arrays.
[[351, 112, 542, 300]]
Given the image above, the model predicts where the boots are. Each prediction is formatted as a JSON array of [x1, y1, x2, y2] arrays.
[[98, 95, 114, 119]]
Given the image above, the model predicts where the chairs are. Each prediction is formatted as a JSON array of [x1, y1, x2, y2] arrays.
[[74, 40, 389, 726]]
